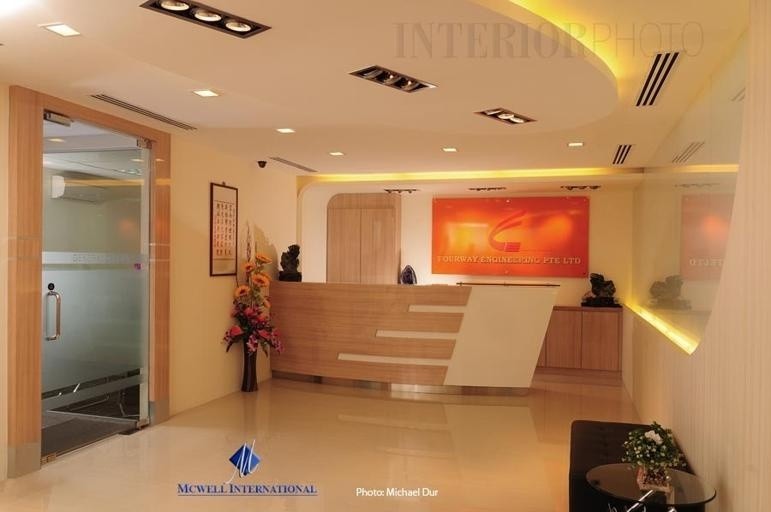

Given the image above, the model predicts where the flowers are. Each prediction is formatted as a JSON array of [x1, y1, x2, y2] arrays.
[[218, 253, 288, 360]]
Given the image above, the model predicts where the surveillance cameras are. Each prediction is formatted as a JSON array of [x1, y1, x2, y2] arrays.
[[257, 161, 266, 167]]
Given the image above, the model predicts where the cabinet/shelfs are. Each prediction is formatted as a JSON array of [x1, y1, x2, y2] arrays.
[[544, 305, 622, 373]]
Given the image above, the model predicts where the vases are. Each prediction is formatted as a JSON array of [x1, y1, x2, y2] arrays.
[[241, 334, 259, 393]]
